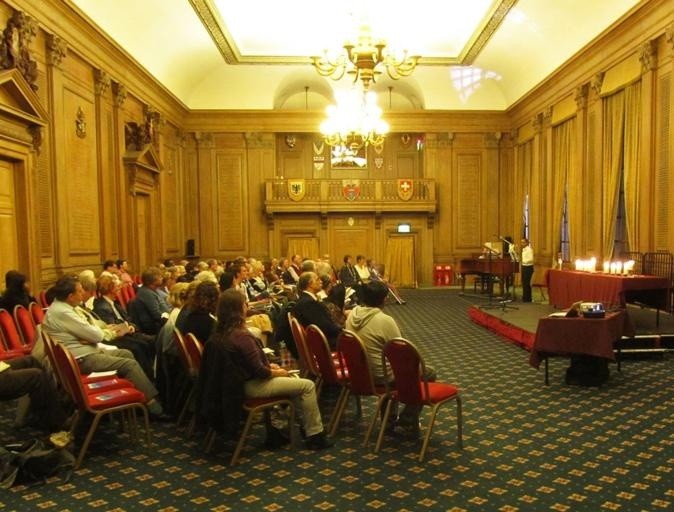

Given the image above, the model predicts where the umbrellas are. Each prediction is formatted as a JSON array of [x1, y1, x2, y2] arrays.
[[148, 410, 176, 423]]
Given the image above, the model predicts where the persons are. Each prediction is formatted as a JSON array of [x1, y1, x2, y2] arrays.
[[0, 355, 76, 433], [45, 287, 154, 382], [42, 273, 164, 415], [518, 239, 535, 302], [209, 288, 337, 451], [46, 254, 383, 364], [292, 271, 343, 351], [345, 280, 438, 437], [1, 269, 37, 318]]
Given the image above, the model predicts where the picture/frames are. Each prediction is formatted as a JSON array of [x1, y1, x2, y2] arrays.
[[542, 268, 674, 330], [528, 310, 625, 387]]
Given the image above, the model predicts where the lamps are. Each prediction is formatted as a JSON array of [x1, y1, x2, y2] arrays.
[[308, 42, 423, 91], [321, 124, 389, 157]]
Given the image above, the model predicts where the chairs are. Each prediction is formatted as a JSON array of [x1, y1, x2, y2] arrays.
[[325, 329, 399, 446], [305, 324, 361, 417], [55, 342, 150, 469], [365, 338, 462, 462], [186, 332, 294, 467], [394, 423, 419, 439], [0, 264, 404, 401], [306, 433, 333, 449]]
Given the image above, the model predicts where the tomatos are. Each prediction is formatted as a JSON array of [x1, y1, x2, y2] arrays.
[[460, 252, 519, 298]]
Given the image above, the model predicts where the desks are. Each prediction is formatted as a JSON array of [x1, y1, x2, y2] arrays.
[[542, 268, 674, 330], [528, 310, 625, 387]]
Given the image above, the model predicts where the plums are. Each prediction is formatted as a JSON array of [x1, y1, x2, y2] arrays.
[[580, 302, 602, 313]]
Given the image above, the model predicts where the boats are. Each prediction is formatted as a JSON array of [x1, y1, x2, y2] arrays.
[[503, 237, 512, 257], [187, 239, 194, 255]]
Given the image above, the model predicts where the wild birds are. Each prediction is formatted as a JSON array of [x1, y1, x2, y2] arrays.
[[549, 301, 581, 318]]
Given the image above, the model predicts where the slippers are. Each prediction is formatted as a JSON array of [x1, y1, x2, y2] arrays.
[[1, 438, 77, 489]]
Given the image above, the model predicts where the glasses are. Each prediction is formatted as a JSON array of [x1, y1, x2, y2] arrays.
[[308, 42, 423, 91], [321, 124, 389, 157]]
